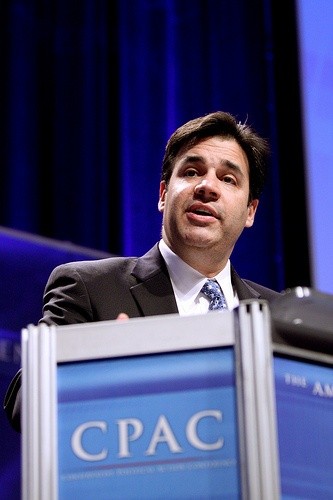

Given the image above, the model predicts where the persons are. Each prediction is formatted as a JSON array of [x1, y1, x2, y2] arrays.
[[2, 109, 284, 443]]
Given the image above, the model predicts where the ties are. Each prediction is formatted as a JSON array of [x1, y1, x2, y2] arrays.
[[200, 278, 228, 309]]
